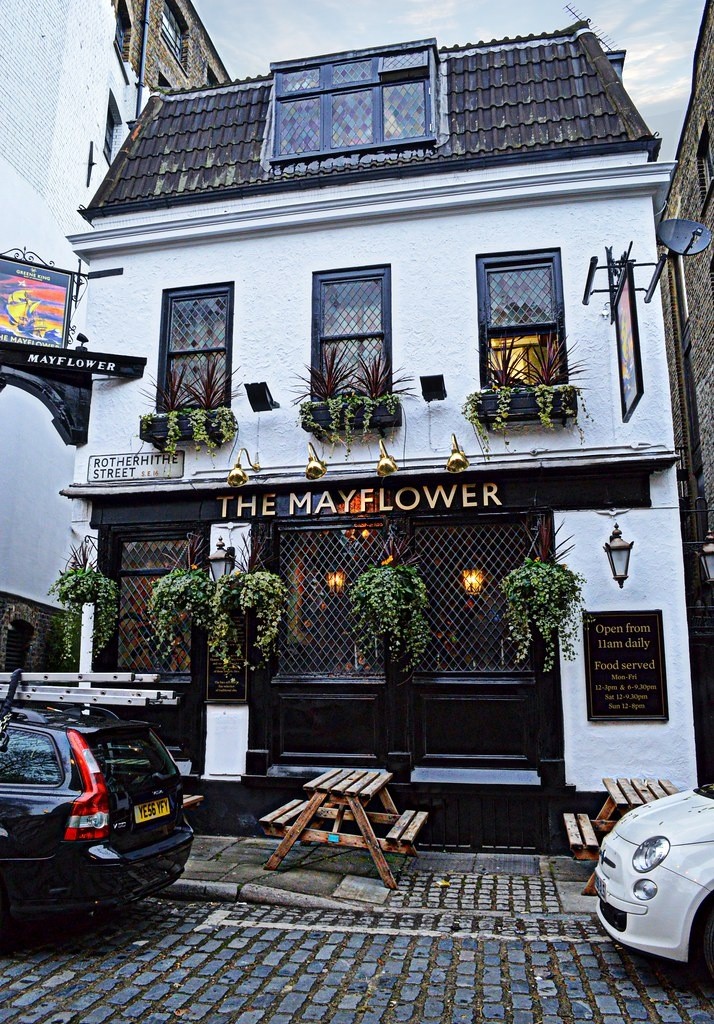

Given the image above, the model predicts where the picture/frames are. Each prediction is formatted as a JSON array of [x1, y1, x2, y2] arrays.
[[0, 254, 75, 350], [612, 261, 645, 424]]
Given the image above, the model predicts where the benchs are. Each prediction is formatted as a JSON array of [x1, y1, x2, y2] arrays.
[[182, 794, 204, 811], [258, 798, 310, 828], [385, 809, 430, 847], [563, 813, 600, 852]]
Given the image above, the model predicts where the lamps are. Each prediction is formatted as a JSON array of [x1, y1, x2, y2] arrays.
[[377, 439, 399, 477], [462, 559, 483, 595], [694, 528, 714, 586], [206, 535, 236, 585], [305, 442, 327, 480], [419, 374, 448, 402], [446, 433, 470, 473], [603, 521, 635, 588], [243, 381, 280, 412], [328, 563, 346, 593], [227, 447, 261, 488]]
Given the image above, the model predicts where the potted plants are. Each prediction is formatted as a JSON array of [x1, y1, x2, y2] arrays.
[[459, 329, 593, 460], [139, 352, 243, 460], [290, 344, 419, 460]]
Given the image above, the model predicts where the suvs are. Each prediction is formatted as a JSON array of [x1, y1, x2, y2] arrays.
[[0, 703, 194, 952]]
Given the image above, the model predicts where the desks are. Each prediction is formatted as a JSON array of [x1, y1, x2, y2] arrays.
[[563, 778, 680, 896], [258, 768, 429, 890]]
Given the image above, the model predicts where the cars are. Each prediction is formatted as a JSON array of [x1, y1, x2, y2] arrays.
[[594, 784, 714, 975]]
